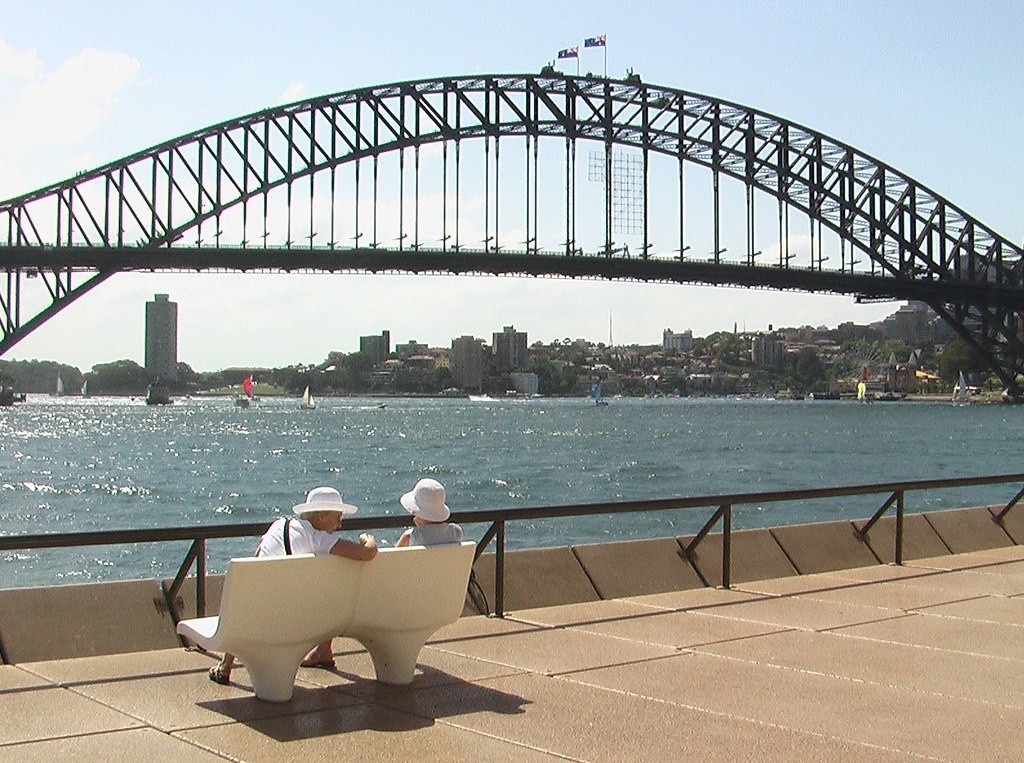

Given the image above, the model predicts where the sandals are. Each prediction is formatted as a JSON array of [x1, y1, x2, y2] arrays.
[[300, 649, 335, 667], [209, 666, 230, 683]]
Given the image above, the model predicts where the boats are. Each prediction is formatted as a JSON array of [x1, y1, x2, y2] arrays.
[[811, 391, 841, 400], [144, 383, 174, 405], [231, 393, 249, 408], [874, 390, 909, 402]]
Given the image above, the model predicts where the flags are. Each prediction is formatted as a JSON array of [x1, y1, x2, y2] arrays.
[[558, 45, 578, 58], [585, 35, 606, 46]]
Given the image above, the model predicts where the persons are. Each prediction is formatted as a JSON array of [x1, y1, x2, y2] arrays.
[[299, 477, 463, 666], [209, 487, 377, 683]]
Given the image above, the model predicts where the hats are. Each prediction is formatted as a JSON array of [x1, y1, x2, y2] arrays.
[[292, 487, 358, 515], [400, 478, 450, 522]]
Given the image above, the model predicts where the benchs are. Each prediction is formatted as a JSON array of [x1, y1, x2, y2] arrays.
[[177, 541, 477, 703]]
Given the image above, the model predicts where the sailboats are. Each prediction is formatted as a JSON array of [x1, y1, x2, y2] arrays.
[[951, 371, 971, 407], [297, 385, 316, 410]]
